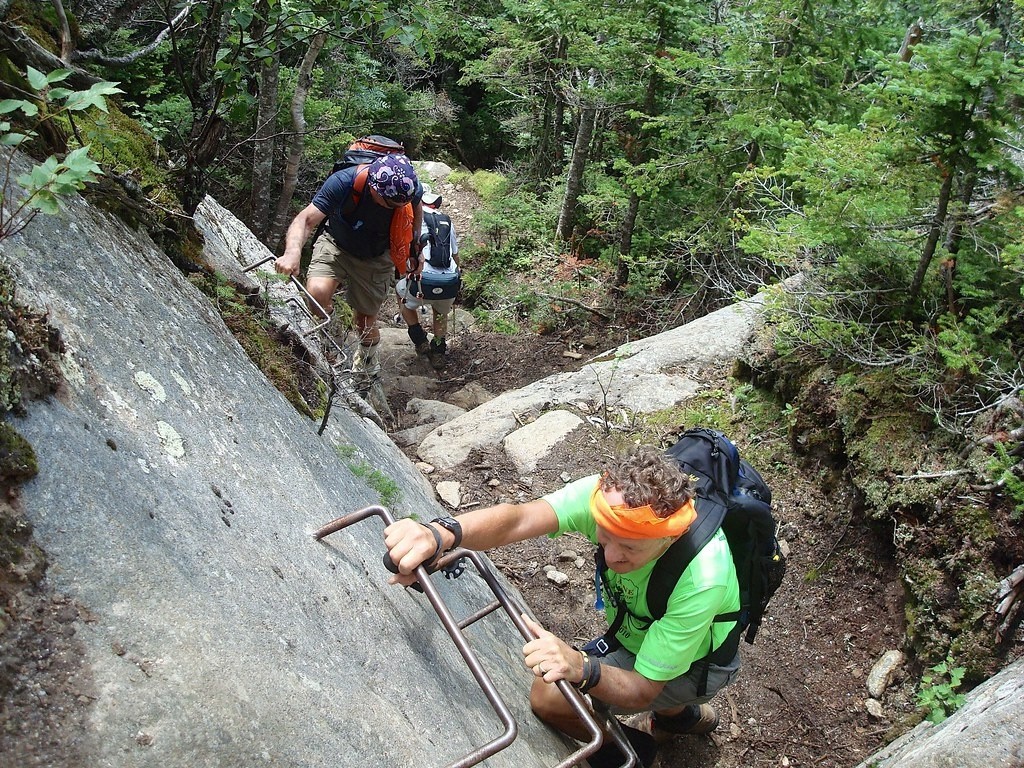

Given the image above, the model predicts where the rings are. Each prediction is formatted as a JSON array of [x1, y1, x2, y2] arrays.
[[538, 663, 547, 675]]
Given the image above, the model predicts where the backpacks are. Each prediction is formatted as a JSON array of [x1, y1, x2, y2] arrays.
[[407, 209, 462, 301], [594, 428, 788, 645], [330, 135, 405, 206]]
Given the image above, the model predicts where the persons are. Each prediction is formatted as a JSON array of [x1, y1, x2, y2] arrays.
[[384, 444, 741, 768], [394, 183, 462, 368], [274, 153, 425, 400]]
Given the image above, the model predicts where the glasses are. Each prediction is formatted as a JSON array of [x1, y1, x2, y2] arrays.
[[382, 197, 397, 210]]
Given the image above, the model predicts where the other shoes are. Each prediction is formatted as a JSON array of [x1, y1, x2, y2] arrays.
[[415, 342, 430, 355], [431, 340, 446, 369], [351, 351, 377, 389]]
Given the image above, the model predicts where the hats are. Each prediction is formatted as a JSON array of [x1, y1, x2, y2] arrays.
[[366, 155, 418, 203], [420, 183, 443, 209]]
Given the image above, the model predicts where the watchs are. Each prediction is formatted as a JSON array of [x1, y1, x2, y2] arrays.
[[430, 516, 462, 553]]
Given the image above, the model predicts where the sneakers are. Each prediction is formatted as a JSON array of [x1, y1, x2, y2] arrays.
[[623, 701, 720, 743]]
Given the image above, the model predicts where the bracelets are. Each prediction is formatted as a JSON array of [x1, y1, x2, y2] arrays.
[[571, 651, 601, 695]]
